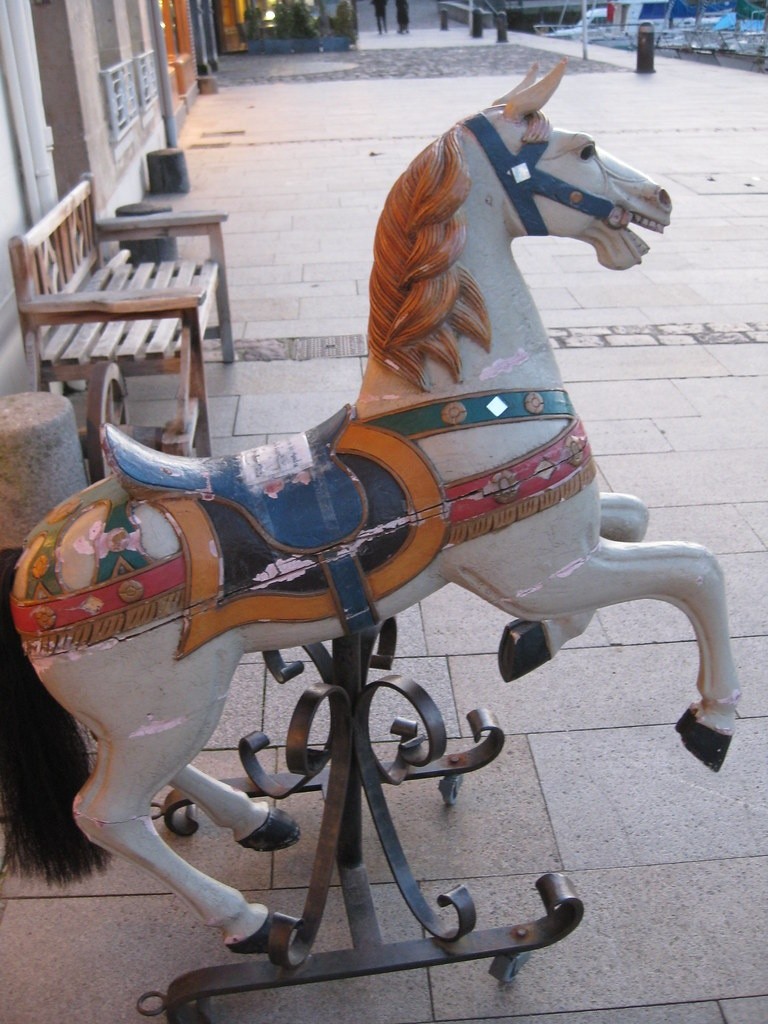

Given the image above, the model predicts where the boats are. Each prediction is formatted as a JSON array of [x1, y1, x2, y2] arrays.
[[531, 0, 768, 54]]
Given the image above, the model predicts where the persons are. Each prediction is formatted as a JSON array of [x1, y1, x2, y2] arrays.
[[371, 0, 388, 35], [396, 0, 409, 35], [605, 0, 616, 30]]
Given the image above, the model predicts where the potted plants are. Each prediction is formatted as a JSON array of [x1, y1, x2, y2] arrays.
[[244, 0, 359, 54], [196, 61, 219, 94]]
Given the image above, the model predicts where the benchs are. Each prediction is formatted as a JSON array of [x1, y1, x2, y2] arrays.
[[9, 174, 235, 459]]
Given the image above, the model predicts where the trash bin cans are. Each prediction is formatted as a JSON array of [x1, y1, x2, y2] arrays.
[[115, 204, 178, 265], [146, 147, 190, 197]]
